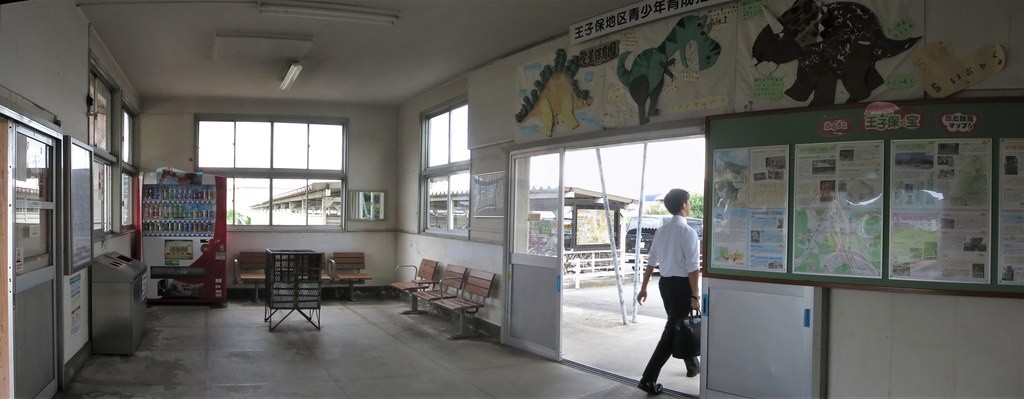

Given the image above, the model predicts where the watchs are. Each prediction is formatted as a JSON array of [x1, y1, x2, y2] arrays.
[[692, 295, 700, 301]]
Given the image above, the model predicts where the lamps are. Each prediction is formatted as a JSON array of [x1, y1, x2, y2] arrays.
[[280, 64, 302, 90], [258, 0, 402, 27]]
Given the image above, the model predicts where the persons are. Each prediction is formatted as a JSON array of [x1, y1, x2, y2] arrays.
[[637, 188, 700, 395]]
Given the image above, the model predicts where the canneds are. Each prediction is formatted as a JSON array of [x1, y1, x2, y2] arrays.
[[192, 189, 213, 200], [183, 209, 212, 217]]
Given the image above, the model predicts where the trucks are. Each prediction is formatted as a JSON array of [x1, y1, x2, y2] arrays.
[[625, 214, 704, 273]]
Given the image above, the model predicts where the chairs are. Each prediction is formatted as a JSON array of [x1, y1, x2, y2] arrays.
[[333, 252, 372, 302], [389, 258, 496, 339]]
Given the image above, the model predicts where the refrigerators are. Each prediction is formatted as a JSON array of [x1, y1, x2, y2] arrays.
[[135, 173, 227, 309]]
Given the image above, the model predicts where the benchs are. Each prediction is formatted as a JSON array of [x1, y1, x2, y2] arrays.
[[231, 251, 336, 304], [569, 258, 627, 290]]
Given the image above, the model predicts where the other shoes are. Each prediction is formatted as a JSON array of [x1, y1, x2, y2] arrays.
[[638, 377, 662, 394], [687, 364, 700, 377]]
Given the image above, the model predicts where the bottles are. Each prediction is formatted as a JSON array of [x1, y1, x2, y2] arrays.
[[142, 184, 216, 238]]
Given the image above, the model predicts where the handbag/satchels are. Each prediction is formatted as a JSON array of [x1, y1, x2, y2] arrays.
[[672, 307, 701, 359]]
